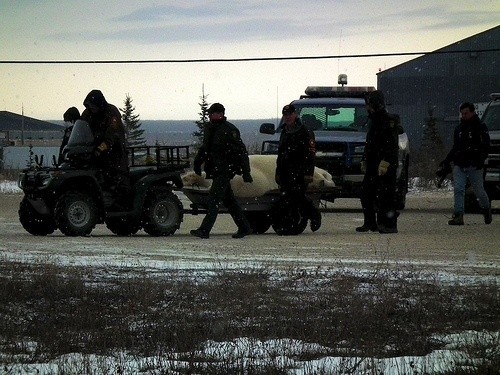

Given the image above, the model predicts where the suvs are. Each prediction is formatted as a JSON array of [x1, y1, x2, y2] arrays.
[[259, 93, 408, 210], [465, 92, 500, 214]]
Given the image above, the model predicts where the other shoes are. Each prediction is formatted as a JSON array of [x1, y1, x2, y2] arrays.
[[310, 214, 321, 232], [281, 228, 298, 235], [482, 207, 492, 224], [449, 214, 464, 225], [232, 228, 250, 238], [378, 224, 397, 233], [356, 222, 377, 231], [190, 229, 209, 239]]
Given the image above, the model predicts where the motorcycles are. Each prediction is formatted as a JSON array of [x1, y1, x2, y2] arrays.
[[17, 119, 190, 236]]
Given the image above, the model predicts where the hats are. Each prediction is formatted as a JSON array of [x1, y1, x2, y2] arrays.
[[282, 105, 295, 115], [206, 103, 224, 113], [368, 91, 384, 105]]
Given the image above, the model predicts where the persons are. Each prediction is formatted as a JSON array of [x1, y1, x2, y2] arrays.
[[58, 107, 80, 164], [387, 112, 409, 217], [356, 92, 398, 234], [274, 104, 322, 236], [190, 103, 253, 239], [79, 90, 130, 211], [447, 101, 492, 226]]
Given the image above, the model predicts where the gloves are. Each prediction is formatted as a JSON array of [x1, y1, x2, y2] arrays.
[[95, 143, 108, 156], [304, 175, 313, 183], [476, 162, 485, 170], [275, 175, 280, 184], [242, 173, 253, 183], [378, 161, 390, 176], [194, 168, 201, 176], [440, 157, 450, 167], [360, 162, 368, 173]]
